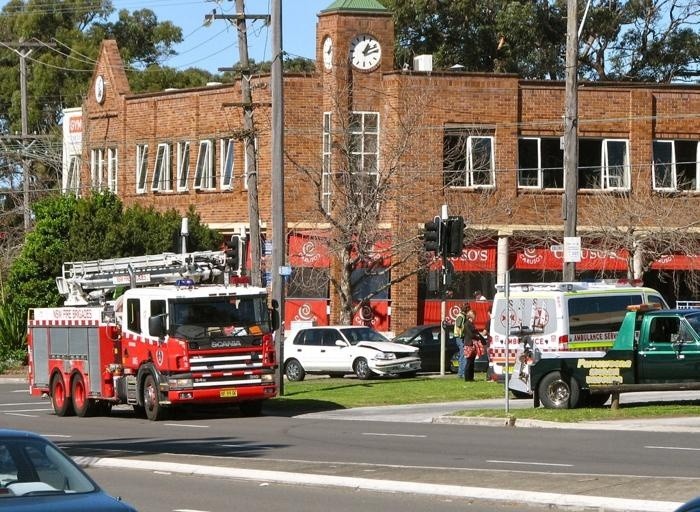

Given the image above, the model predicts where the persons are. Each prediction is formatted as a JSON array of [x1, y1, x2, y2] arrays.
[[462, 309, 487, 382], [444, 287, 455, 299], [454, 302, 471, 378], [481, 306, 492, 336], [473, 289, 487, 301]]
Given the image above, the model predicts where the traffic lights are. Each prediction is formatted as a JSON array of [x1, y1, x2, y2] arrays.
[[224, 234, 244, 273], [422, 215, 444, 253], [447, 213, 467, 259]]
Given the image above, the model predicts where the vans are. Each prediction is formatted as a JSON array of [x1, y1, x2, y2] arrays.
[[486, 280, 674, 399]]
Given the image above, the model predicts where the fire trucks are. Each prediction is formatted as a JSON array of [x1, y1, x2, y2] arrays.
[[22, 246, 280, 422]]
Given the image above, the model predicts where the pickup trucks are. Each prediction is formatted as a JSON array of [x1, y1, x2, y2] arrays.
[[527, 302, 700, 411]]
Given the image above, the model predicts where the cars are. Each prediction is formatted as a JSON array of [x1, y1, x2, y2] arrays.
[[0, 427, 140, 512], [388, 321, 481, 374], [282, 323, 423, 383]]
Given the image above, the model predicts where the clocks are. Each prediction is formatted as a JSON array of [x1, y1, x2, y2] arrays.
[[94, 75, 106, 105], [349, 34, 382, 72], [321, 37, 333, 72]]
